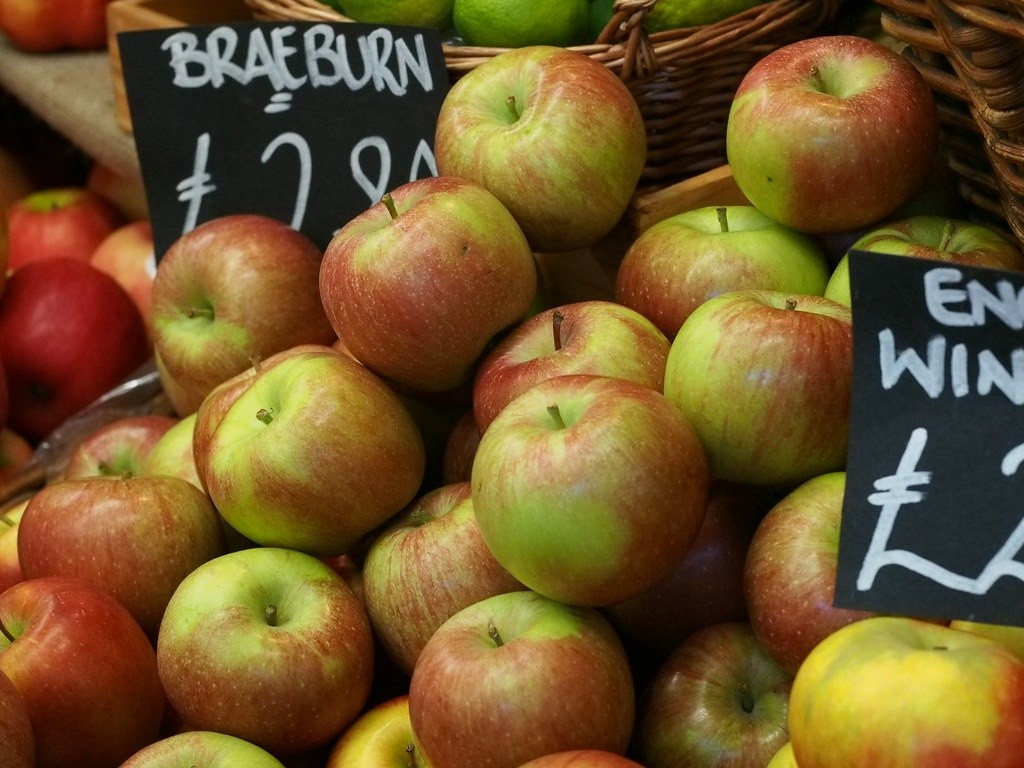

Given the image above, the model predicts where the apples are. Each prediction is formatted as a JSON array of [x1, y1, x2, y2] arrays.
[[0, 0, 1024, 768]]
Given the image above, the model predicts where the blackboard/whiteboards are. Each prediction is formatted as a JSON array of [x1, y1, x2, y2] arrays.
[[832, 248, 1024, 631], [116, 23, 451, 272]]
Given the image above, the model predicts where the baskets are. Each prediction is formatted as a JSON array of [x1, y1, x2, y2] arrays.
[[869, 0, 1024, 247], [247, 0, 836, 192]]
